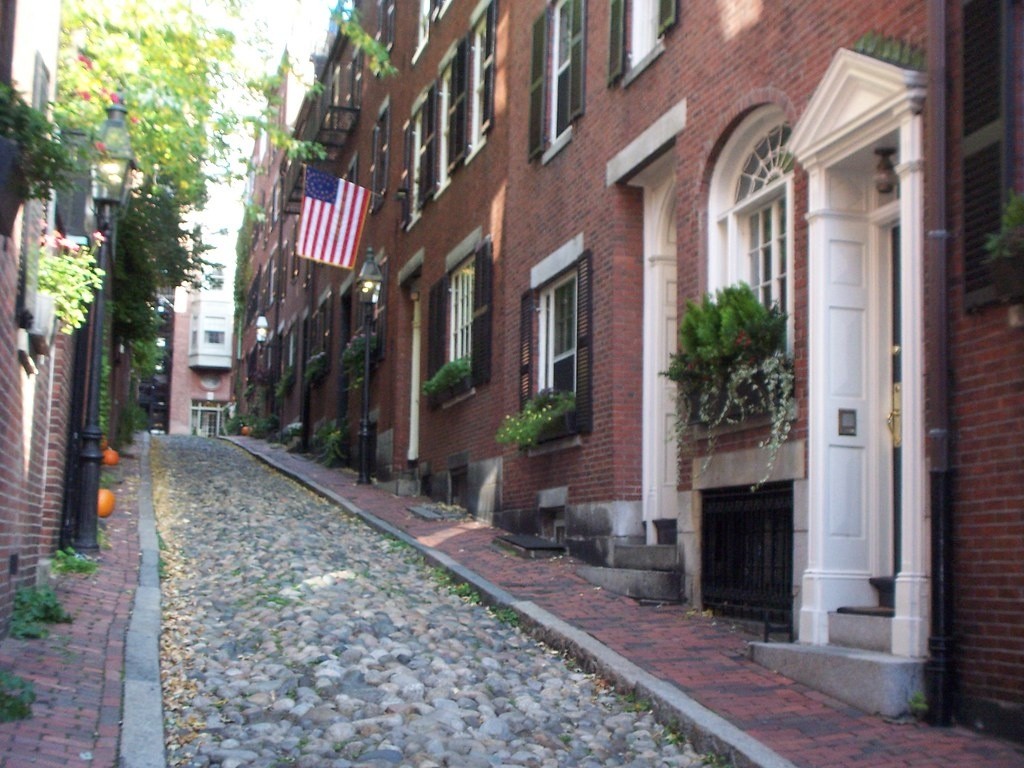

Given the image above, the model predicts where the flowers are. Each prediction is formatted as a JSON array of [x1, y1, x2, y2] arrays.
[[303, 351, 330, 389], [658, 281, 797, 494], [341, 333, 378, 395], [497, 387, 579, 457], [25, 219, 107, 336]]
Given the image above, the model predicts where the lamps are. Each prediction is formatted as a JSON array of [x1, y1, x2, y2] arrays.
[[875, 145, 899, 192]]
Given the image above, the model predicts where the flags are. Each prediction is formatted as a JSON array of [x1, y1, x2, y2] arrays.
[[295, 164, 372, 270]]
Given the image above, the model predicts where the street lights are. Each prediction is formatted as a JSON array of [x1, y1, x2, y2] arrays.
[[356, 246, 385, 485], [59, 83, 138, 556]]
